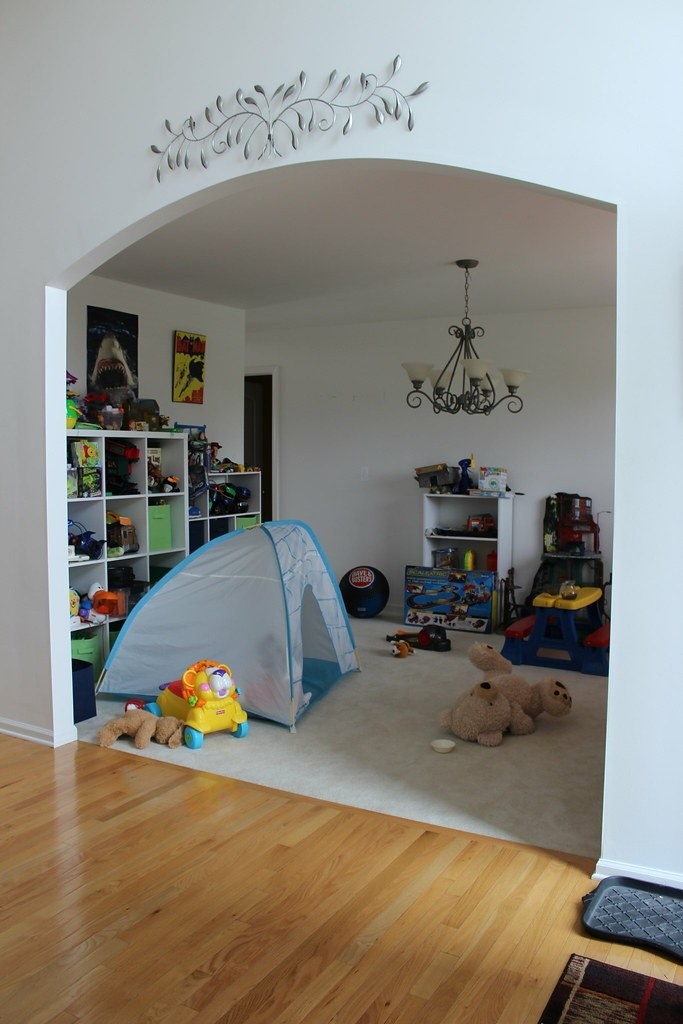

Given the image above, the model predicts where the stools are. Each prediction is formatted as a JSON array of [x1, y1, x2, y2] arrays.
[[520, 582, 607, 670], [523, 548, 610, 621]]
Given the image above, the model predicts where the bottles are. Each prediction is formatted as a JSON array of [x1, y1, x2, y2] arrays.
[[486, 550, 497, 572], [464, 550, 473, 570]]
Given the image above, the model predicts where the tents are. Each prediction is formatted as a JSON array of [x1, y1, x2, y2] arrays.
[[93, 520, 362, 733]]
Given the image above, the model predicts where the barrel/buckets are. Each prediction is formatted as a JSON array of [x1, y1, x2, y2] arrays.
[[435, 548, 453, 568]]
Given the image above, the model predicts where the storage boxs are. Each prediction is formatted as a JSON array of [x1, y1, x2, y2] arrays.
[[405, 565, 495, 633], [478, 467, 508, 492]]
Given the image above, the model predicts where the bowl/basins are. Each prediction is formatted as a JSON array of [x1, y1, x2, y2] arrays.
[[430, 739, 455, 753]]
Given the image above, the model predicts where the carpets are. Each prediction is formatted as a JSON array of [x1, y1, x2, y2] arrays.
[[536, 952, 683, 1024], [76, 615, 607, 860]]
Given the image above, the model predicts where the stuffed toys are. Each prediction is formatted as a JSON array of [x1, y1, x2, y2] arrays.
[[98, 709, 185, 749], [390, 640, 416, 658], [439, 642, 572, 746]]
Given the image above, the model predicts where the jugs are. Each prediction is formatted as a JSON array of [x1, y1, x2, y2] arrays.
[[560, 580, 577, 600]]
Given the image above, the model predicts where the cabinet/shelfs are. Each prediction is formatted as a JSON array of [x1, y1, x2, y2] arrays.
[[66, 429, 262, 724], [420, 492, 538, 625]]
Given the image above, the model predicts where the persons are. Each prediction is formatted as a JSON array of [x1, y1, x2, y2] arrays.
[[209, 441, 222, 470]]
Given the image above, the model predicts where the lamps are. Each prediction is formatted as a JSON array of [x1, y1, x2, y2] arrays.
[[402, 258, 529, 415]]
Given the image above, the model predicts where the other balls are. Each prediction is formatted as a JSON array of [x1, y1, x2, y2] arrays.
[[339, 565, 391, 618]]
